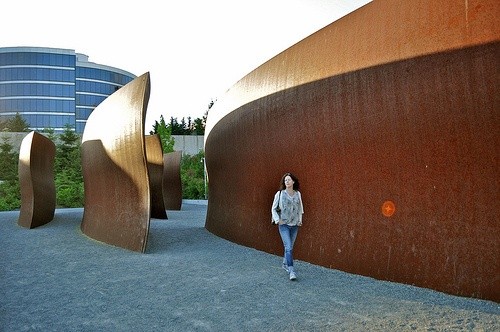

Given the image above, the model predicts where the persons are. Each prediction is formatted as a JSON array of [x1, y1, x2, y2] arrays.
[[272, 173, 304, 280]]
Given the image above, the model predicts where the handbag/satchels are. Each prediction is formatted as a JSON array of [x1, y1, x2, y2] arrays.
[[272, 190, 281, 225]]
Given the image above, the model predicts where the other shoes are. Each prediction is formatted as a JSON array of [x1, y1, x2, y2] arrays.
[[282, 263, 290, 271], [289, 269, 297, 279]]
